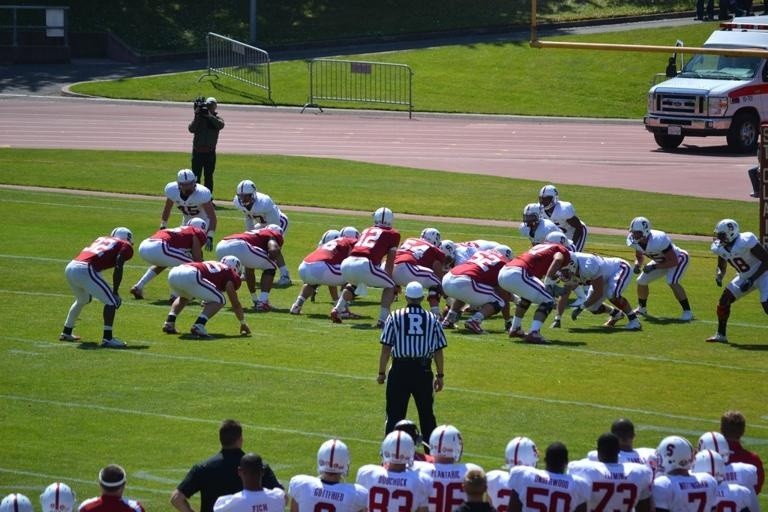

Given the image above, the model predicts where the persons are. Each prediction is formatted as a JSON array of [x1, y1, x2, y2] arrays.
[[131, 218, 208, 304], [161, 169, 217, 261], [213, 453, 285, 512], [422, 422, 495, 511], [60, 227, 134, 348], [163, 255, 251, 338], [290, 436, 369, 512], [216, 223, 290, 311], [354, 430, 433, 511], [508, 442, 590, 512], [565, 432, 653, 512], [376, 281, 447, 455], [0, 493, 33, 512], [170, 419, 288, 512], [77, 464, 145, 512], [687, 411, 761, 512], [483, 433, 540, 512], [648, 427, 719, 512], [388, 419, 435, 468], [233, 180, 291, 288], [290, 184, 768, 343], [40, 482, 76, 512], [585, 416, 662, 512], [189, 97, 224, 207]]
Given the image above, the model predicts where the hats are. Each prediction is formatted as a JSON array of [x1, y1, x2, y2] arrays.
[[406, 281, 424, 299], [205, 96, 216, 106]]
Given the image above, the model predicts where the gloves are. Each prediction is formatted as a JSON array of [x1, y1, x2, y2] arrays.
[[740, 278, 752, 292], [632, 265, 654, 274], [111, 292, 121, 309], [204, 237, 213, 251], [714, 274, 724, 288]]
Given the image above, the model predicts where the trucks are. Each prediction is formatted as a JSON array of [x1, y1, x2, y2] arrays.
[[639, 14, 768, 150]]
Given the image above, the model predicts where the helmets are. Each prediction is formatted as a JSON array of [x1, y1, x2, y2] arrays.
[[112, 227, 132, 242], [381, 420, 463, 466], [711, 218, 740, 247], [177, 168, 196, 184], [559, 252, 579, 281], [321, 207, 393, 244], [187, 216, 208, 234], [0, 481, 77, 512], [236, 180, 256, 195], [421, 228, 512, 260], [317, 439, 350, 476], [221, 255, 242, 273], [656, 431, 733, 480], [505, 436, 538, 466], [265, 224, 284, 235], [629, 216, 651, 244], [522, 184, 569, 248]]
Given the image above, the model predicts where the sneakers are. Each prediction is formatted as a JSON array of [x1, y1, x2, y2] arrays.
[[436, 305, 642, 344], [102, 339, 126, 347], [704, 331, 727, 343], [130, 286, 143, 299], [341, 311, 360, 318], [633, 307, 648, 315], [332, 310, 339, 323], [277, 276, 291, 286], [679, 310, 693, 321], [192, 325, 211, 339], [168, 296, 178, 303], [162, 323, 180, 335], [289, 305, 297, 313], [59, 333, 81, 341], [238, 272, 246, 280], [377, 319, 384, 329], [251, 301, 271, 312]]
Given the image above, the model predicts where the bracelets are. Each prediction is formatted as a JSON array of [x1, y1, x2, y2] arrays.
[[240, 320, 246, 325], [379, 373, 385, 375], [435, 374, 444, 377]]
